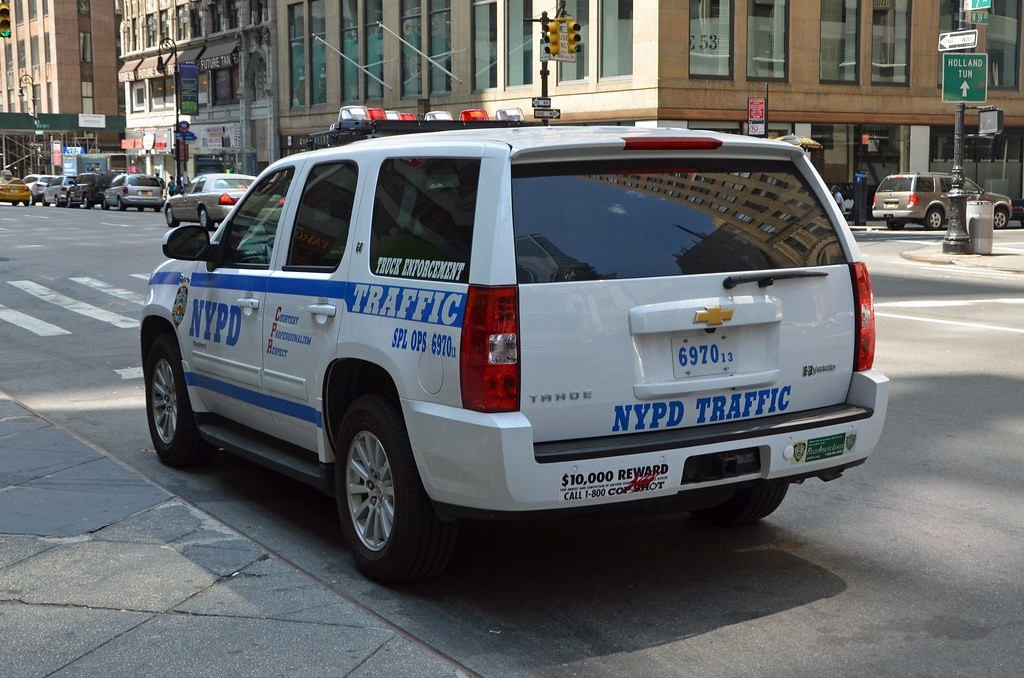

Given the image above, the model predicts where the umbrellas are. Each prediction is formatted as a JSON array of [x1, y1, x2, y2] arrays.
[[775, 133, 823, 152]]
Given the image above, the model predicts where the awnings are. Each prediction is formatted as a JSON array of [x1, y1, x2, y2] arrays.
[[118, 41, 239, 82]]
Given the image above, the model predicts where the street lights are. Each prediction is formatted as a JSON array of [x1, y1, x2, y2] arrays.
[[156, 37, 183, 193], [18, 73, 39, 174]]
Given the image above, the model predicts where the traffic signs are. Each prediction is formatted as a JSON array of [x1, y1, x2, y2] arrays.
[[942, 53, 986, 102], [532, 97, 560, 119], [938, 29, 977, 50]]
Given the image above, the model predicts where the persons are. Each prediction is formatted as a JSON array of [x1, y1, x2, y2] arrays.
[[831, 185, 845, 214], [88, 165, 101, 173], [156, 173, 184, 197]]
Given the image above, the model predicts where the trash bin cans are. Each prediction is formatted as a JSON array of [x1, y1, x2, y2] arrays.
[[966, 200, 994, 255]]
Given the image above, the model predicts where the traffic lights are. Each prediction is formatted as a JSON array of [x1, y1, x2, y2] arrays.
[[568, 21, 581, 55], [0, 4, 11, 38], [544, 23, 558, 55]]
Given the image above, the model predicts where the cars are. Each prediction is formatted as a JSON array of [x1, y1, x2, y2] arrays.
[[0, 170, 30, 206], [1011, 199, 1024, 229], [165, 174, 257, 231], [42, 175, 78, 207]]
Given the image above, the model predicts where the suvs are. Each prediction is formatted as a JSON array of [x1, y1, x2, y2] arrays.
[[139, 105, 890, 589], [101, 173, 164, 213], [21, 174, 52, 206], [872, 172, 1012, 231], [66, 172, 116, 209]]
